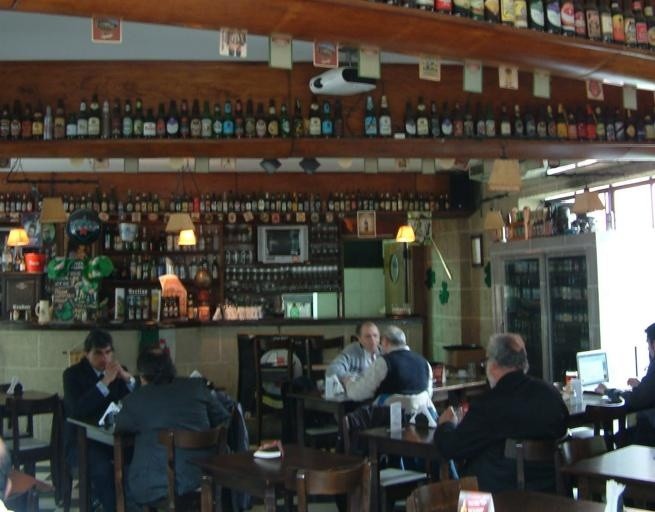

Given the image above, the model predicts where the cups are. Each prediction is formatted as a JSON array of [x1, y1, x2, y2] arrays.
[[35, 300, 50, 323], [570, 378, 583, 400]]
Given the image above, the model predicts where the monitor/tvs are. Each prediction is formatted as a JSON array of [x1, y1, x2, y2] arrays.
[[257, 225, 308, 266]]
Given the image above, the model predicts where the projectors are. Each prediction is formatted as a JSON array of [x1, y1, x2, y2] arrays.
[[309, 66, 376, 96]]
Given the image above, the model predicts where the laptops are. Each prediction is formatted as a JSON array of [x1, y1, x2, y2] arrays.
[[576, 350, 611, 395]]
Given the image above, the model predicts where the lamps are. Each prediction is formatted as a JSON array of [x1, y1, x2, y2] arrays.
[[164, 213, 196, 231], [394, 223, 417, 243], [176, 229, 198, 246], [487, 159, 522, 192], [5, 225, 31, 247], [483, 210, 505, 230], [569, 184, 606, 214], [38, 196, 66, 223]]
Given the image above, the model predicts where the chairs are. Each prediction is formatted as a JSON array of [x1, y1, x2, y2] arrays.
[[0, 333, 654, 511]]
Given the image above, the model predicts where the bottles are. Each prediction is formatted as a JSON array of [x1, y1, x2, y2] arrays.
[[402, 93, 653, 143], [507, 255, 590, 377], [19, 259, 26, 272], [290, 301, 300, 319], [3, 94, 336, 136], [505, 205, 571, 239], [386, 1, 655, 52], [14, 250, 21, 271], [364, 86, 395, 137], [225, 248, 341, 296], [0, 189, 460, 253], [126, 290, 264, 319], [130, 254, 220, 282], [1, 245, 13, 272]]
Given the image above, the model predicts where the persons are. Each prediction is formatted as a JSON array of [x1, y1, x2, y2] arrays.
[[325, 319, 384, 454], [433, 332, 574, 499], [63, 332, 142, 512], [341, 324, 433, 455], [0, 438, 15, 512], [595, 322, 655, 448], [113, 346, 229, 512]]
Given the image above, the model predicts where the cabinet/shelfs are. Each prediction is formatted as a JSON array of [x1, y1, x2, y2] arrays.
[[0, 209, 220, 287]]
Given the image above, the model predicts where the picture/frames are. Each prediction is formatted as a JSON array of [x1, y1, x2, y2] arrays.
[[356, 210, 377, 239], [470, 232, 483, 268]]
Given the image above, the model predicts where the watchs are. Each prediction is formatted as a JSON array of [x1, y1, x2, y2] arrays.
[[126, 376, 136, 386]]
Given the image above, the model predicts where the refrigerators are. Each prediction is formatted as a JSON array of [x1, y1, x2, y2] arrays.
[[490, 229, 645, 384]]
